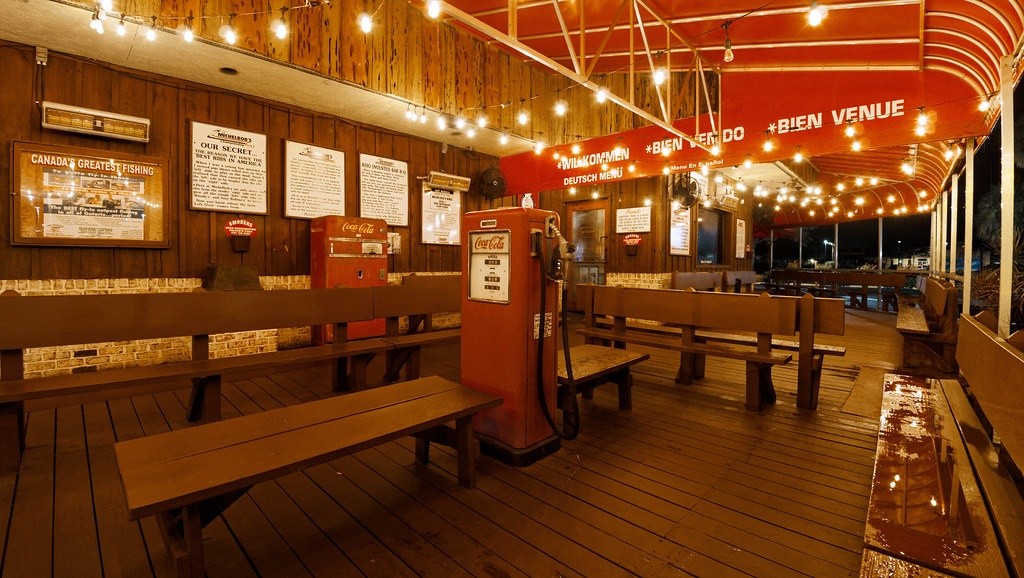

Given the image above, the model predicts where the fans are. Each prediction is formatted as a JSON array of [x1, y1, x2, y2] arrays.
[[481, 168, 507, 199], [672, 177, 702, 208]]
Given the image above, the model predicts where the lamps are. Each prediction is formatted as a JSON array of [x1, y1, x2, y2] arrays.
[[39, 101, 151, 145], [722, 187, 740, 209], [429, 171, 471, 193]]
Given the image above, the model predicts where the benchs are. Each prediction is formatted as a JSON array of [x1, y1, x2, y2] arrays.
[[1, 268, 1024, 578]]
